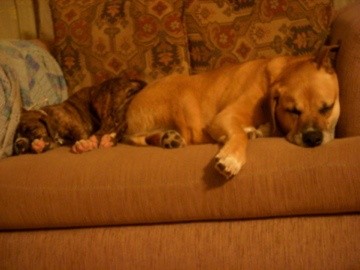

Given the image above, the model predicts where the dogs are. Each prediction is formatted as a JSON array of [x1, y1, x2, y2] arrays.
[[12, 75, 146, 157], [119, 42, 341, 180]]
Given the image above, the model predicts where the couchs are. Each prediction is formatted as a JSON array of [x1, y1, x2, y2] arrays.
[[0, 0, 360, 269]]
